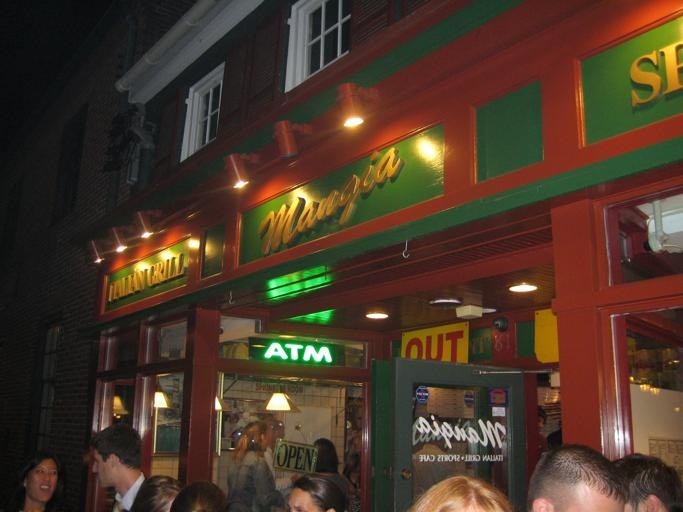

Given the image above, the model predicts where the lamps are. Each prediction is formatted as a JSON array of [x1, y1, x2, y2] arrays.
[[273, 120, 312, 157], [113, 395, 130, 420], [135, 209, 162, 239], [365, 303, 391, 320], [505, 275, 542, 295], [215, 396, 233, 414], [223, 153, 260, 189], [109, 225, 134, 252], [266, 382, 292, 411], [335, 81, 370, 129], [89, 239, 111, 264], [154, 391, 172, 409], [429, 292, 465, 310]]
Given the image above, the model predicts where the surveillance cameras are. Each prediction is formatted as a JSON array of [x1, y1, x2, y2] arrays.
[[493, 317, 508, 331]]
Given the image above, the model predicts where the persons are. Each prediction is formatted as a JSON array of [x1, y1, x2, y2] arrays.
[[412, 411, 467, 500], [606, 452, 683, 511], [89, 421, 147, 512], [0, 447, 63, 511], [401, 472, 515, 511], [257, 412, 301, 492], [128, 475, 184, 512], [300, 436, 353, 512], [282, 471, 349, 512], [168, 480, 227, 511], [538, 404, 548, 435], [226, 420, 289, 511], [339, 424, 361, 511], [523, 442, 630, 511], [544, 427, 562, 449]]
